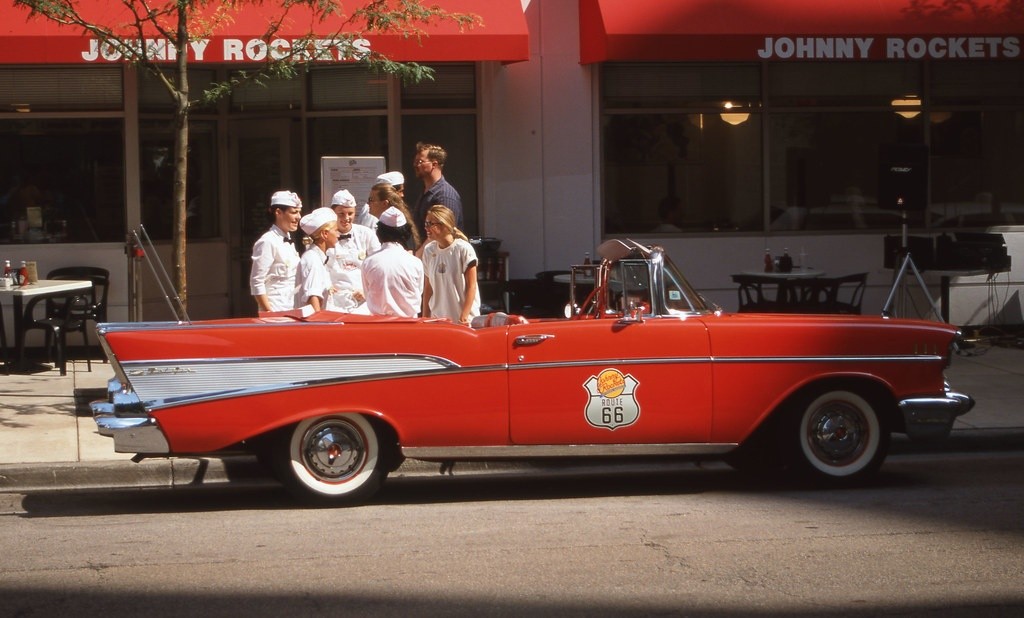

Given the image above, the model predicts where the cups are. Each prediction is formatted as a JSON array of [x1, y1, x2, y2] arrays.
[[19, 220, 26, 234], [774, 256, 784, 273]]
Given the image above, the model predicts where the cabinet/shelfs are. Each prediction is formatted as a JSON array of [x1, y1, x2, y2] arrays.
[[479, 251, 511, 314]]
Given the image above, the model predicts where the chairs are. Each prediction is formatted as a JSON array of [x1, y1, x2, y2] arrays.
[[775, 279, 838, 314], [471, 312, 529, 329], [44, 266, 109, 364], [817, 272, 868, 314], [731, 274, 776, 313], [15, 278, 98, 376]]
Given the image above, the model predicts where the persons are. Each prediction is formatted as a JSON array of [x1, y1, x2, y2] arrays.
[[412, 141, 463, 244], [245, 171, 428, 319], [653, 197, 686, 235], [420, 205, 481, 327]]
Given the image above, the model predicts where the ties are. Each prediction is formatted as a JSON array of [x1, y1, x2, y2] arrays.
[[283, 237, 294, 244]]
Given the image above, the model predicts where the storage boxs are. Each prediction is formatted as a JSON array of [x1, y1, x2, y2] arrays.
[[775, 255, 792, 272], [0, 277, 14, 287]]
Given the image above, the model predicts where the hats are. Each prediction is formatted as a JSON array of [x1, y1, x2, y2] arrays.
[[299, 206, 337, 236], [377, 170, 404, 185], [270, 190, 302, 208], [380, 206, 406, 227], [331, 189, 357, 208]]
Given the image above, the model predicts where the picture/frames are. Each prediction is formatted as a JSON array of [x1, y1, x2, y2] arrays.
[[604, 113, 704, 167]]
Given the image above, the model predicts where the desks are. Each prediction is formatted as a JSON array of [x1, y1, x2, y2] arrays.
[[0, 280, 93, 375], [744, 271, 827, 278], [552, 273, 615, 284], [905, 267, 1011, 348]]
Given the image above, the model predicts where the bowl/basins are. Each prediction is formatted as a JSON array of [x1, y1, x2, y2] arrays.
[[0, 278, 13, 286]]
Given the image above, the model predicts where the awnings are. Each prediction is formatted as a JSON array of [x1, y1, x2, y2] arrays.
[[0, 0, 531, 68], [580, 0, 1024, 65]]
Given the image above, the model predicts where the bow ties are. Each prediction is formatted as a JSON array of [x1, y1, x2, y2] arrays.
[[337, 234, 351, 240], [325, 256, 329, 264]]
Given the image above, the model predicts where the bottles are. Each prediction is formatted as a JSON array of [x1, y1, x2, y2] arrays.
[[800, 253, 809, 272], [783, 248, 792, 272], [5, 260, 11, 274], [764, 249, 772, 273], [583, 252, 593, 278], [18, 261, 29, 286]]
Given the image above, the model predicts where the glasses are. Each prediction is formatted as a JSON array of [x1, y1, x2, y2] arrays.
[[368, 196, 383, 202], [425, 221, 442, 227], [414, 159, 434, 165]]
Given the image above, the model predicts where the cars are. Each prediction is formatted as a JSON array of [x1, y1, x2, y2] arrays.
[[89, 237, 973, 506]]
